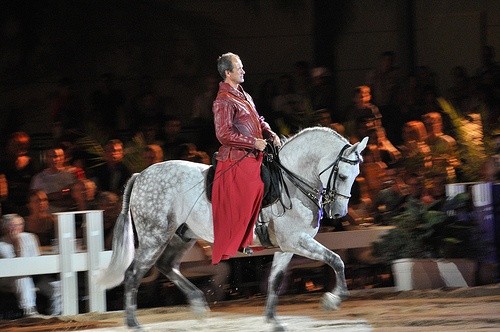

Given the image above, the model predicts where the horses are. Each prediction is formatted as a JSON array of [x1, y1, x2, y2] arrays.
[[95, 127, 372, 331]]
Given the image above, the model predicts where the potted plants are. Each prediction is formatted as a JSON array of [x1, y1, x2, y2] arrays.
[[372, 97, 500, 291]]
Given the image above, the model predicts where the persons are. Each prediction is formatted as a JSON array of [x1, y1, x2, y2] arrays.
[[211, 52, 281, 264], [0, 85, 499, 317]]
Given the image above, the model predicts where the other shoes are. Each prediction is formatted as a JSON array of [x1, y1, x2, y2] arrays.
[[298, 279, 323, 292], [239, 246, 252, 253], [24, 307, 39, 316], [211, 282, 240, 299]]
